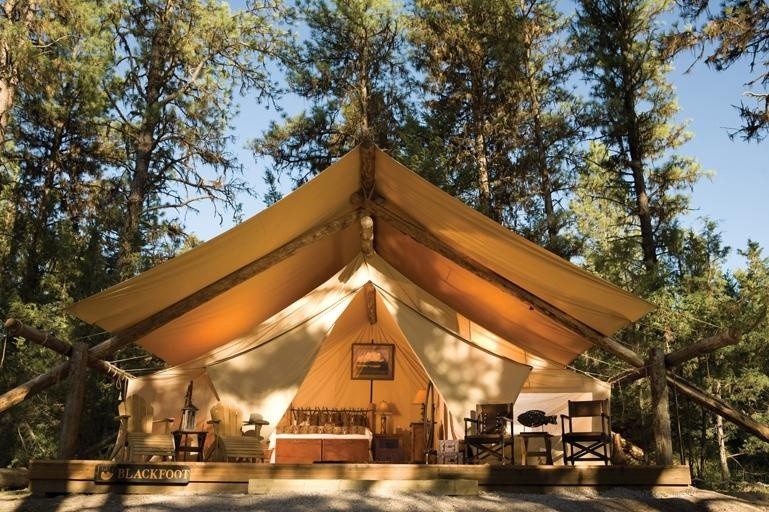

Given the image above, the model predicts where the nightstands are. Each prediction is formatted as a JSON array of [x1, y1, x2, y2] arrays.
[[372, 433, 402, 463]]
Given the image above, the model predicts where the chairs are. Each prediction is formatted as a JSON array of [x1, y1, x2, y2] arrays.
[[204, 399, 269, 463], [110, 393, 178, 462], [463, 402, 514, 466], [559, 398, 615, 467]]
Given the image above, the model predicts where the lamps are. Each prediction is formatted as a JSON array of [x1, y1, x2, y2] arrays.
[[373, 399, 393, 435], [410, 390, 428, 422]]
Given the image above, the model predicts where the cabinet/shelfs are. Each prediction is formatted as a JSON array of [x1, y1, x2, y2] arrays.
[[408, 422, 437, 463]]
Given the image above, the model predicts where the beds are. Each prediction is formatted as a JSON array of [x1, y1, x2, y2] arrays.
[[274, 403, 374, 464]]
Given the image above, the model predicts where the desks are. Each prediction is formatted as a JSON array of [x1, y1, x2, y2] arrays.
[[517, 434, 555, 466], [170, 430, 209, 461]]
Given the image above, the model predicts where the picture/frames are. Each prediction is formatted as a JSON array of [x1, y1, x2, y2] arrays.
[[351, 342, 395, 381]]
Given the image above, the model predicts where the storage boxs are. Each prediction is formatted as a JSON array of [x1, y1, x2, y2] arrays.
[[437, 439, 462, 464]]
